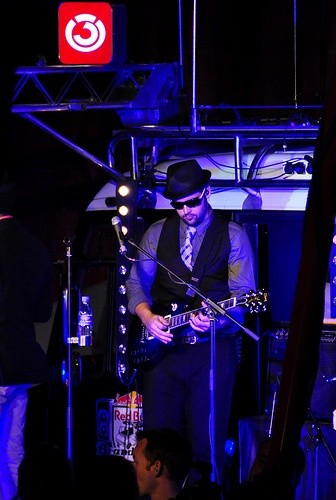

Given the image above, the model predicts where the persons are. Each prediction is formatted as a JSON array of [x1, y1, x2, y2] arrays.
[[0, 171, 56, 500], [125, 159, 258, 477], [131, 427, 197, 500]]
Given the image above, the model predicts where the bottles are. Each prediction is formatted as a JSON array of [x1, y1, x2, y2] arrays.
[[77, 309, 94, 347]]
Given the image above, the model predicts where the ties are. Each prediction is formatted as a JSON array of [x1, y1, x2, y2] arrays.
[[180, 226, 196, 272]]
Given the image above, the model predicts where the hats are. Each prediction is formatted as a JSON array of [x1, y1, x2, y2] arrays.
[[162, 160, 211, 200]]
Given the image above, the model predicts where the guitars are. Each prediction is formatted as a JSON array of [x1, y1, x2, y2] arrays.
[[127, 288, 268, 372]]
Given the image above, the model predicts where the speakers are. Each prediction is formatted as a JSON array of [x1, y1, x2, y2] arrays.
[[264, 331, 336, 421], [235, 211, 334, 332], [238, 420, 336, 499]]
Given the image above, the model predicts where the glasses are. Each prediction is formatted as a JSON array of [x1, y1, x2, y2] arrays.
[[170, 188, 206, 210]]
